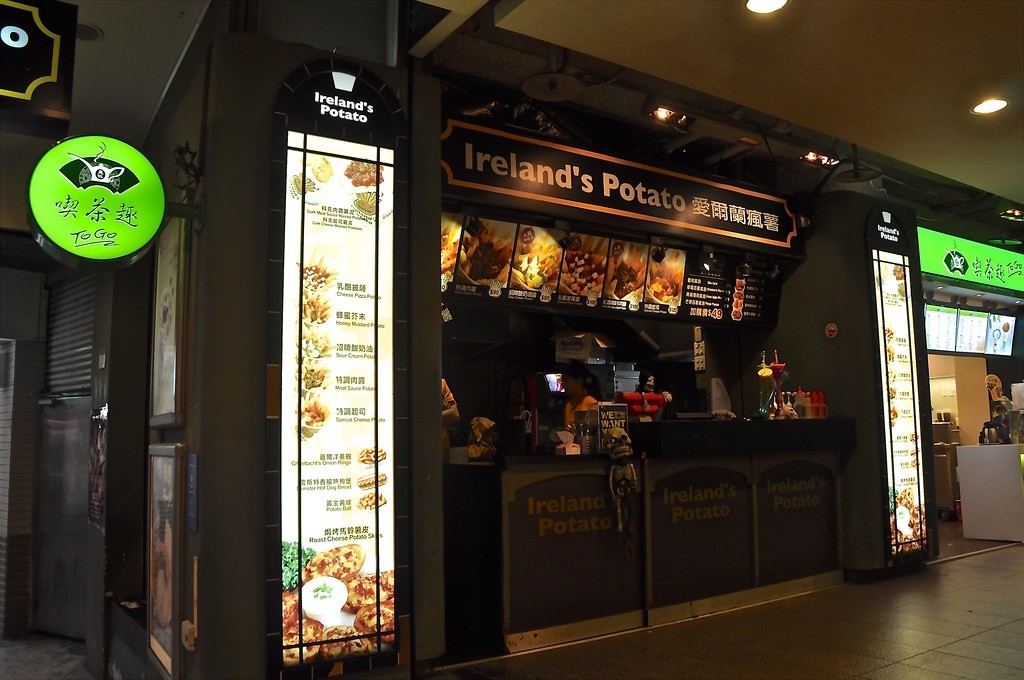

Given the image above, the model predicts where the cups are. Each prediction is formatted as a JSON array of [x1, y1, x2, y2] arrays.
[[574, 410, 598, 454]]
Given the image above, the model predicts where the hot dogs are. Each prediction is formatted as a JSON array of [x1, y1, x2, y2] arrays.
[[357, 474, 388, 489]]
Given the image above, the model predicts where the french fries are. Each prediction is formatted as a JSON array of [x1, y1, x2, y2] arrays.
[[295, 253, 341, 420], [440, 213, 684, 303]]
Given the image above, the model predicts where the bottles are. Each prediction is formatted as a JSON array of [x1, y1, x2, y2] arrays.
[[769, 386, 828, 421]]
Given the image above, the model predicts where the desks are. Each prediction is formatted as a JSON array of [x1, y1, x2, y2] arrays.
[[956, 444, 1024, 542]]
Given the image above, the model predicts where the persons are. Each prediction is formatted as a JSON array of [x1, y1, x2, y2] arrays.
[[560, 359, 605, 430], [441, 378, 459, 463]]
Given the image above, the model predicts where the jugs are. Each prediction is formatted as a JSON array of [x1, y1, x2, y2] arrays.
[[982, 421, 1000, 444]]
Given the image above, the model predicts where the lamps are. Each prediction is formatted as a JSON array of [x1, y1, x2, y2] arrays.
[[810, 156, 881, 198], [640, 93, 840, 174], [559, 231, 574, 250], [651, 242, 669, 263], [499, 72, 585, 123], [465, 219, 481, 237]]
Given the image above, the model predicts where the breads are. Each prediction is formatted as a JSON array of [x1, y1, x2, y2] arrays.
[[282, 544, 395, 666], [888, 488, 928, 553]]
